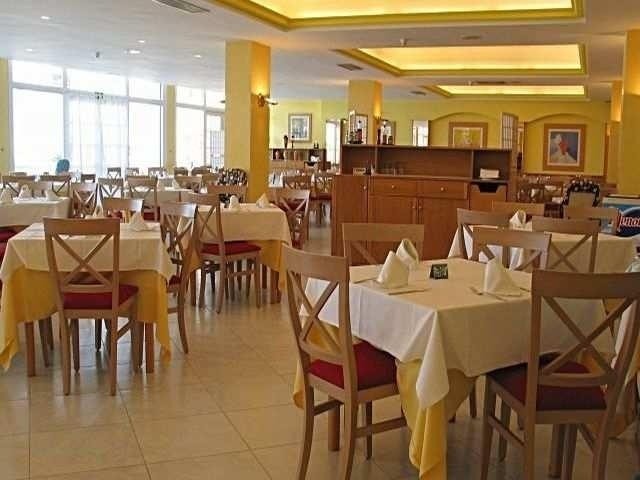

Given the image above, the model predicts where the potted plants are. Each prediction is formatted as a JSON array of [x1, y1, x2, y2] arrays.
[[541, 122, 588, 174], [446, 121, 491, 149], [354, 112, 369, 145], [287, 111, 314, 144]]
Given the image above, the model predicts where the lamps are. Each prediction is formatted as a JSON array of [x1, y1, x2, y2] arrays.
[[373, 111, 382, 126], [255, 92, 280, 109]]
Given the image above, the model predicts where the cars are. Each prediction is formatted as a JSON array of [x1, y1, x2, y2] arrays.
[[230, 195, 239, 208], [224, 199, 229, 208]]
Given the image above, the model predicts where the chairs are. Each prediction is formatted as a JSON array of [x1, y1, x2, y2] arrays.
[[188, 192, 262, 313], [282, 242, 400, 480], [483, 269, 640, 478], [131, 202, 199, 364], [341, 173, 640, 273], [0, 150, 333, 305], [43, 214, 120, 396]]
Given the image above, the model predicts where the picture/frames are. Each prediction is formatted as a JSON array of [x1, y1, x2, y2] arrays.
[[354, 112, 369, 145], [446, 121, 491, 149], [287, 111, 314, 144], [541, 122, 588, 174]]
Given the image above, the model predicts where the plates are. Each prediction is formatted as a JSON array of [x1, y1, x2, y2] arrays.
[[266, 146, 327, 172], [327, 140, 523, 270]]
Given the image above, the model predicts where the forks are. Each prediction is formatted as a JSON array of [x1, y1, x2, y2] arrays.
[[471, 284, 506, 305]]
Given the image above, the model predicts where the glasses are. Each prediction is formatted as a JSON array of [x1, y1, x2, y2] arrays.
[[373, 111, 382, 126], [255, 92, 280, 109]]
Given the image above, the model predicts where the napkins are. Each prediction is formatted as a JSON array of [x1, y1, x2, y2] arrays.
[[395, 238, 422, 273], [478, 258, 523, 298], [376, 251, 408, 288]]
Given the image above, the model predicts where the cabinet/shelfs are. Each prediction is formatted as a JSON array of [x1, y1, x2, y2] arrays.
[[294, 151, 298, 161], [283, 135, 288, 149], [276, 150, 280, 160], [344, 118, 362, 144], [376, 119, 393, 144], [266, 146, 327, 172], [327, 140, 523, 270]]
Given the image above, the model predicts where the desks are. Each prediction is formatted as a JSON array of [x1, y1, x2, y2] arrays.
[[2, 222, 173, 377], [292, 257, 615, 479]]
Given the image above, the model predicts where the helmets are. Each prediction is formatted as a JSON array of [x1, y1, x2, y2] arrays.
[[129, 225, 159, 232], [352, 274, 428, 295]]
